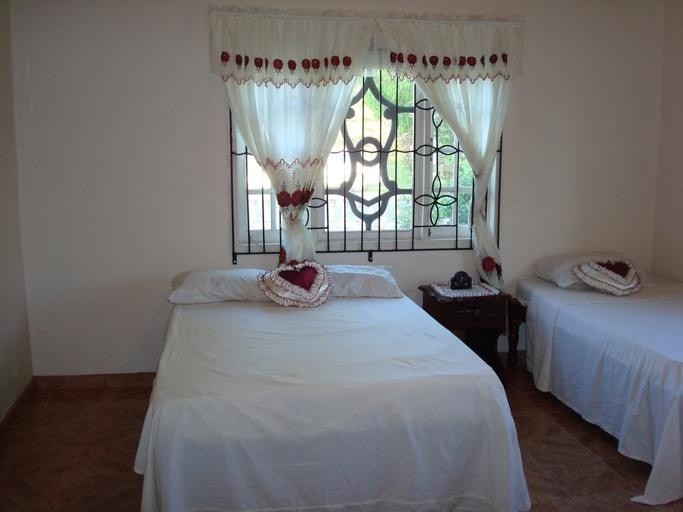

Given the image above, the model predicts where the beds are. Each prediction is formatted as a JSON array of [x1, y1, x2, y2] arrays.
[[515, 277, 683, 500], [143, 288, 520, 511]]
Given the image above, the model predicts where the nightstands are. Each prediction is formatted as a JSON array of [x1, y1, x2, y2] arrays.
[[416, 283, 507, 387]]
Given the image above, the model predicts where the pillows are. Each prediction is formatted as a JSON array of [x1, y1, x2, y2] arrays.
[[536, 250, 648, 296], [165, 259, 404, 307]]
